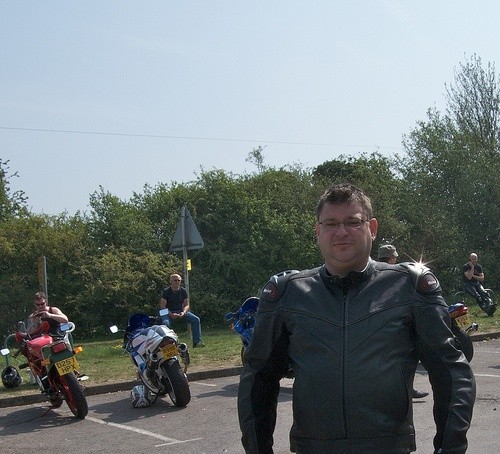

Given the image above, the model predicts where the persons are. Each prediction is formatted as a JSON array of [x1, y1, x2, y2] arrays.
[[161, 274, 206, 349], [28, 293, 68, 336], [237, 183, 476, 454], [378, 244, 429, 398], [462, 253, 493, 310]]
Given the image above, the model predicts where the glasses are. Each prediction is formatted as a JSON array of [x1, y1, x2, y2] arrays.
[[35, 302, 45, 306], [319, 218, 369, 229], [173, 280, 181, 281]]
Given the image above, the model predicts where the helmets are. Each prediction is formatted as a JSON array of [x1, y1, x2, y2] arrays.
[[131, 385, 151, 408], [2, 366, 21, 388]]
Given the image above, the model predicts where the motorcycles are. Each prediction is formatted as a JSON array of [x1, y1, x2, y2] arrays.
[[445, 303, 479, 363], [224, 296, 257, 370], [109, 308, 192, 408], [12, 314, 90, 419]]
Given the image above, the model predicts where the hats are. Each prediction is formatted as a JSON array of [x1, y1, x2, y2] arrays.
[[378, 245, 399, 258]]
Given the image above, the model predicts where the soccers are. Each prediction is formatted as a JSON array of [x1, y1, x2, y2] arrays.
[[131, 385, 151, 408]]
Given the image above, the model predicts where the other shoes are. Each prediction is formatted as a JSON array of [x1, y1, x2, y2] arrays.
[[413, 389, 428, 398], [193, 343, 205, 348], [487, 306, 496, 314]]
